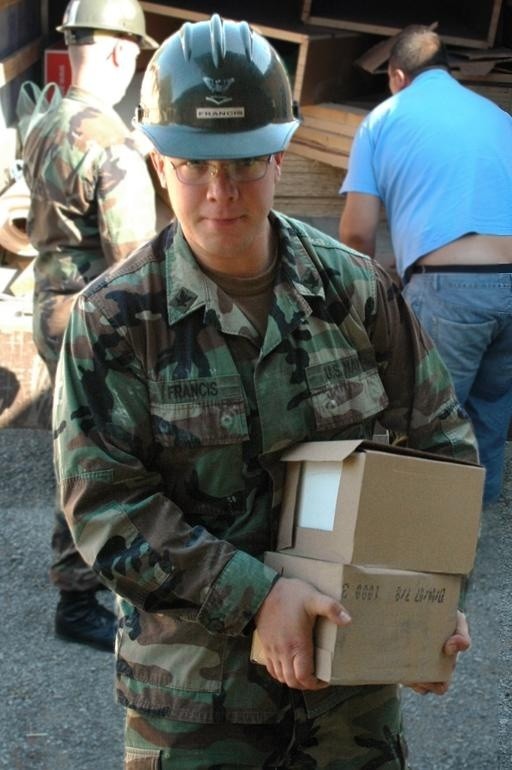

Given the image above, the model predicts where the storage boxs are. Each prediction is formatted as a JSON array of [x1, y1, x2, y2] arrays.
[[275, 438, 486, 577], [250, 547, 462, 685]]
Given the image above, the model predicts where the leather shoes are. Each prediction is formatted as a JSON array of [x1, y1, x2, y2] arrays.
[[53, 585, 118, 653]]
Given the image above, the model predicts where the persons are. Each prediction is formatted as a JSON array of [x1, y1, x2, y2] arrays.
[[48, 14, 479, 768], [339, 28, 512, 512], [22, 0, 158, 653]]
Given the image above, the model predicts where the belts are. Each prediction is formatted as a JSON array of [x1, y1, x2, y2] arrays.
[[411, 263, 511, 273]]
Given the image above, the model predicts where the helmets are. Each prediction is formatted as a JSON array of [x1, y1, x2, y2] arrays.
[[131, 13, 304, 159], [55, 0, 160, 51]]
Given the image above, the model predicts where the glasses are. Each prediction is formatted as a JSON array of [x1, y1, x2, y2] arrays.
[[162, 154, 274, 184]]
